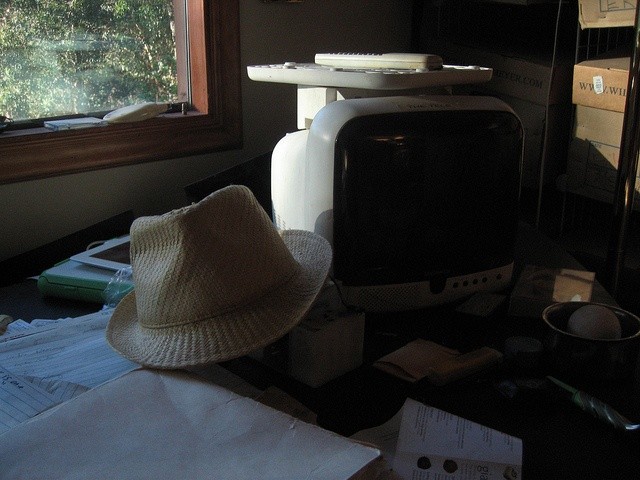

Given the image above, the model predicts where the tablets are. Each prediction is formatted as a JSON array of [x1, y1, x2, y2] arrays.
[[70, 235, 131, 275]]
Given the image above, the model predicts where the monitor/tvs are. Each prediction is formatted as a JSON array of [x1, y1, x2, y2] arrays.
[[271, 96, 524, 312]]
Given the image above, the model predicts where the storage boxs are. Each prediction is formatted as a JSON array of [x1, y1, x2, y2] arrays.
[[578, 0, 637, 31], [570, 56, 640, 211], [425, 32, 569, 189]]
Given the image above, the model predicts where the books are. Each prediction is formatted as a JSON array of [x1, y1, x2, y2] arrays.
[[0, 365, 383, 480]]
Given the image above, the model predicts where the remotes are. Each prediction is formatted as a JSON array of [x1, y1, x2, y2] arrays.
[[315, 53, 443, 69]]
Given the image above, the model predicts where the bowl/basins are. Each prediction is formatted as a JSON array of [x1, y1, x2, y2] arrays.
[[538, 298, 639, 366]]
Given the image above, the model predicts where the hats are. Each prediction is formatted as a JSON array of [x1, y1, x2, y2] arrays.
[[106, 186, 333, 369]]
